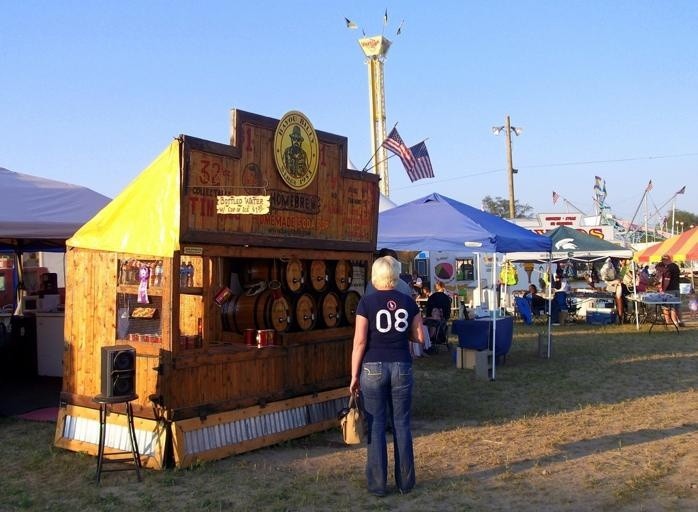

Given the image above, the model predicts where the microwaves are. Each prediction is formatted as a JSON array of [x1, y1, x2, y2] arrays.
[[22, 294, 60, 314]]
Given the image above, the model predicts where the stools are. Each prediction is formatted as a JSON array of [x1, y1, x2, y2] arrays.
[[93, 393, 141, 485]]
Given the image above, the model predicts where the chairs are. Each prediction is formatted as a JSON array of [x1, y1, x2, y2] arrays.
[[423, 293, 450, 353], [554, 291, 577, 317], [512, 290, 534, 324]]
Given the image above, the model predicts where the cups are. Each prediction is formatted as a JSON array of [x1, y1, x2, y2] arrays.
[[216, 286, 234, 306], [56, 303, 64, 312], [244, 327, 275, 347], [129, 332, 202, 353]]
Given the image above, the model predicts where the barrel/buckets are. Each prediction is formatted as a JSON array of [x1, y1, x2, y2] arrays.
[[223, 259, 361, 332]]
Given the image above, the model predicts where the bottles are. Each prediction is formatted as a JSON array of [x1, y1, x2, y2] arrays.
[[496, 305, 500, 317], [475, 305, 481, 319], [121, 258, 194, 289]]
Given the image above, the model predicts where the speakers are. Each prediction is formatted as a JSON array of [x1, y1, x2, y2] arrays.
[[101, 345, 136, 397]]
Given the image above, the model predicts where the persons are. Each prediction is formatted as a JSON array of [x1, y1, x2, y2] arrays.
[[362, 248, 416, 308], [282, 126, 309, 177], [408, 274, 449, 312], [348, 254, 426, 495], [518, 255, 685, 328]]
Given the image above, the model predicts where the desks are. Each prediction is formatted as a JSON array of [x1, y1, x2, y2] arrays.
[[537, 293, 615, 299], [36, 313, 64, 379], [626, 296, 682, 334], [453, 315, 513, 369]]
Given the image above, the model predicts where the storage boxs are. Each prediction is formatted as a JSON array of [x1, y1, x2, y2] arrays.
[[586, 308, 616, 325]]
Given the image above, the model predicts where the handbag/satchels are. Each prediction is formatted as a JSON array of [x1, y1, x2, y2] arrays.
[[340, 392, 366, 444]]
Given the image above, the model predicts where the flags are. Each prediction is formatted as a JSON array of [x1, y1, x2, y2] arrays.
[[397, 24, 403, 35], [676, 188, 686, 195], [346, 19, 358, 30], [383, 10, 388, 24], [402, 142, 435, 183], [382, 127, 415, 174], [552, 191, 559, 204], [647, 181, 655, 192]]
